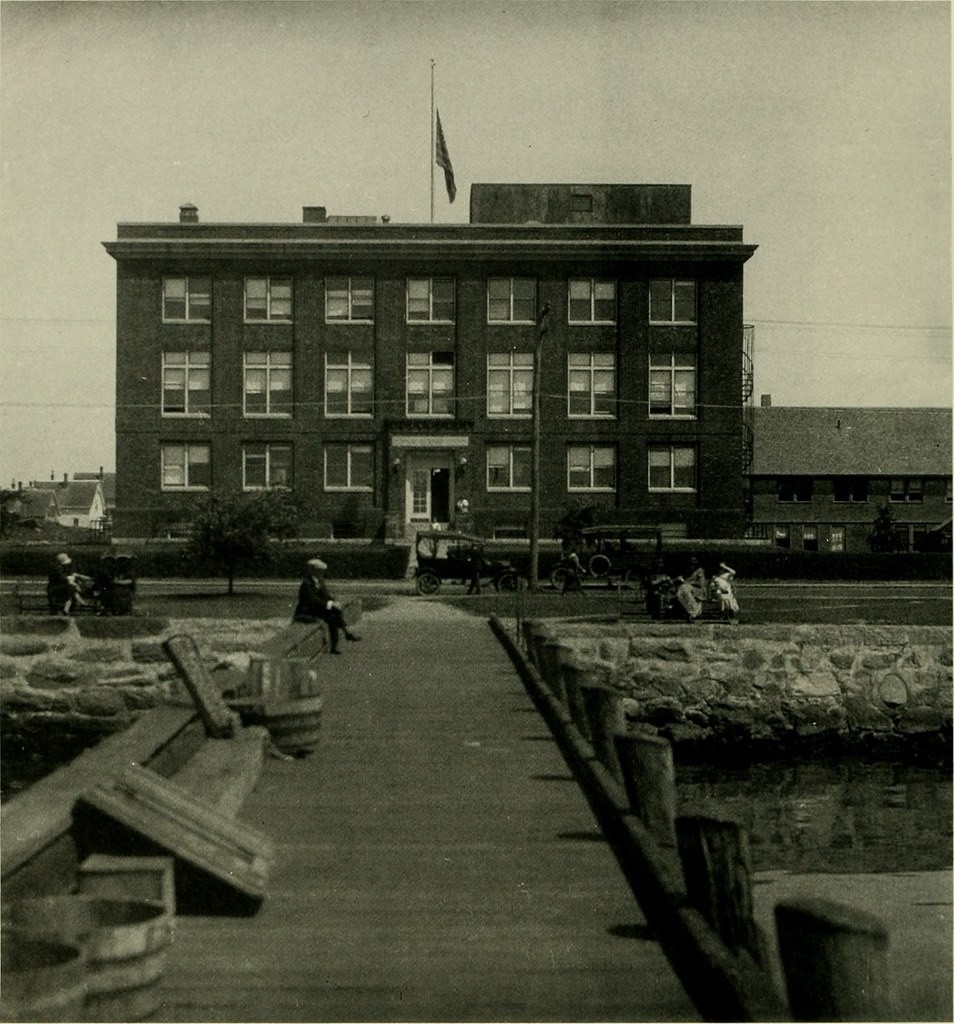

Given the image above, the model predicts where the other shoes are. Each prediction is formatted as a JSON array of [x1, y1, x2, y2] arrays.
[[346, 633, 361, 641], [324, 639, 340, 654]]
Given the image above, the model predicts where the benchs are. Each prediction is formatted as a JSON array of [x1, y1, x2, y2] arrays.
[[14, 579, 137, 614], [647, 586, 724, 620]]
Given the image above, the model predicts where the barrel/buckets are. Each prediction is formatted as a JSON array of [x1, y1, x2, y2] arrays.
[[0, 924, 92, 1024], [242, 693, 321, 756], [1, 896, 174, 1023]]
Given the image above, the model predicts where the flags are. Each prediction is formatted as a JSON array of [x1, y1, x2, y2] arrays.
[[435, 108, 458, 204]]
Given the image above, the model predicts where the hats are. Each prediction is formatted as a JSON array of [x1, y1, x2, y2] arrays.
[[56, 553, 71, 565], [308, 558, 327, 569]]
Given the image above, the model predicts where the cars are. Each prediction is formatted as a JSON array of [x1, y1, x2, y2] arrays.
[[412, 530, 521, 597], [550, 524, 664, 590]]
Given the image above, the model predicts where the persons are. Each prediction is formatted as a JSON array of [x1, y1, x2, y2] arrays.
[[560, 540, 586, 596], [466, 544, 484, 595], [429, 517, 442, 544], [677, 557, 708, 617], [707, 563, 739, 617], [47, 553, 94, 615], [294, 559, 363, 654], [456, 495, 470, 513]]
